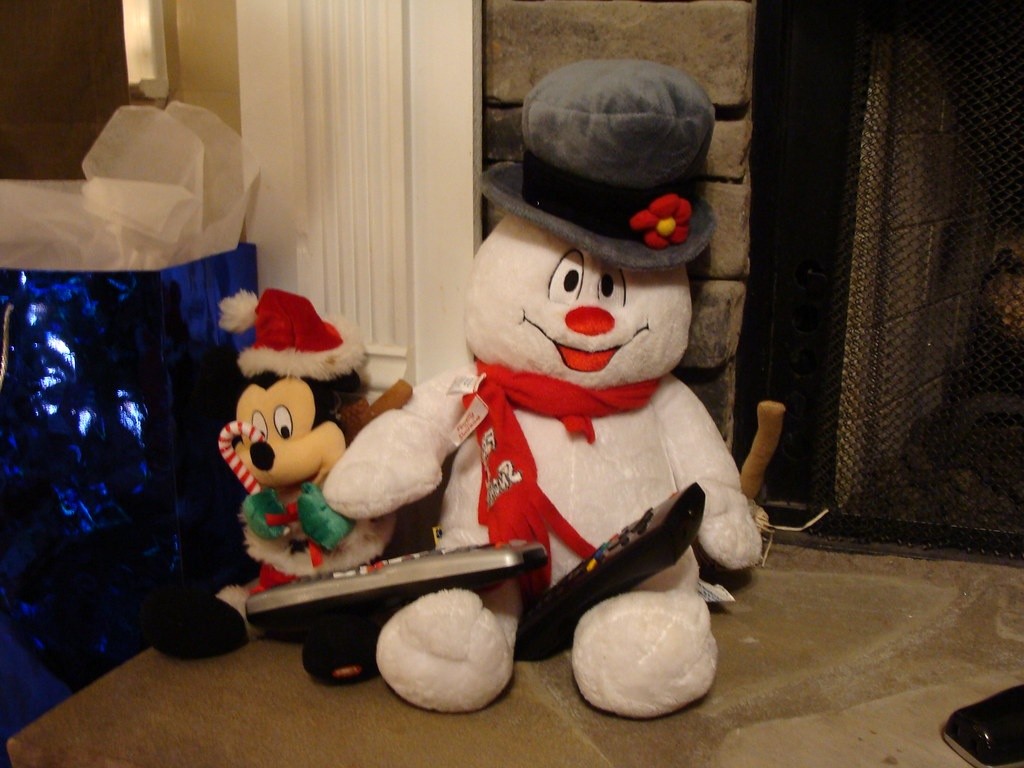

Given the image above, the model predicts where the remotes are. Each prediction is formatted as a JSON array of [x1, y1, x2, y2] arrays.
[[245, 540, 549, 634], [515, 481, 705, 663]]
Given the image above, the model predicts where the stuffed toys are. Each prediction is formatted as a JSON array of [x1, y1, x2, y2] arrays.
[[324, 51, 763, 718], [140, 288, 411, 684]]
